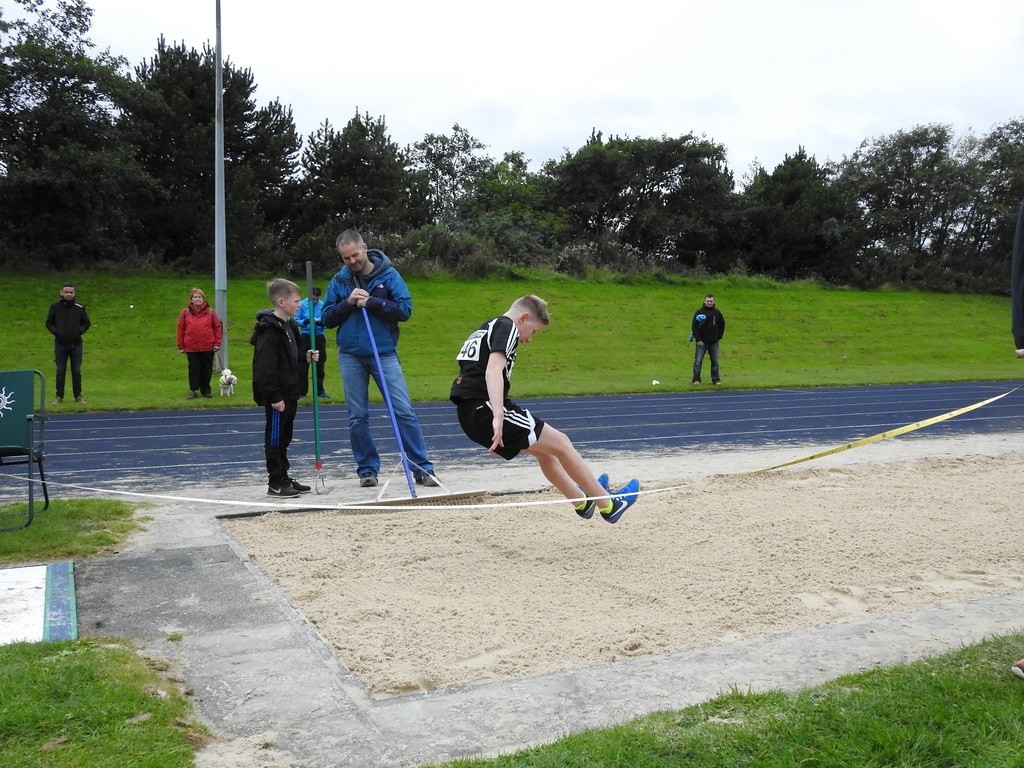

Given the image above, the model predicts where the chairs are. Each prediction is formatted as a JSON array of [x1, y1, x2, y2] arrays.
[[0, 369, 49, 532]]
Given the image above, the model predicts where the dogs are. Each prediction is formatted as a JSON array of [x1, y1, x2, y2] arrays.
[[219, 368, 237, 396]]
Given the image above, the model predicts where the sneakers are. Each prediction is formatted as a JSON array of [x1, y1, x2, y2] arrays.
[[600, 479, 640, 524], [266, 478, 311, 498], [360, 472, 378, 486], [574, 473, 609, 519], [416, 470, 442, 487]]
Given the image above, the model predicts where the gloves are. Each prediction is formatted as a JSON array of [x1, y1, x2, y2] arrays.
[[303, 319, 310, 324], [314, 321, 327, 326]]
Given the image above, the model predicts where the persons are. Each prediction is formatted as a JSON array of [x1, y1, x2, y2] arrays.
[[692, 294, 726, 386], [46, 283, 91, 406], [177, 287, 223, 400], [321, 229, 442, 487], [449, 294, 639, 523], [294, 287, 331, 398], [250, 278, 319, 499]]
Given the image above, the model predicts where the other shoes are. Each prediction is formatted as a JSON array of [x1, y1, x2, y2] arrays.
[[52, 397, 63, 404], [319, 393, 330, 398], [75, 396, 87, 403], [188, 393, 197, 399], [694, 381, 700, 384], [715, 382, 721, 385], [203, 393, 213, 398]]
